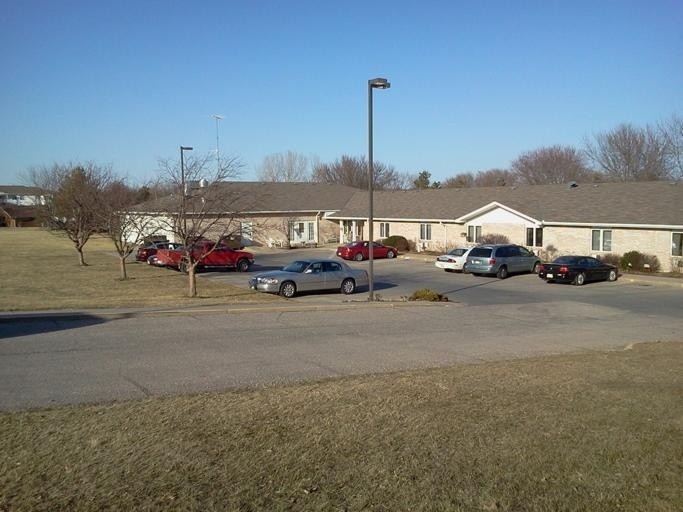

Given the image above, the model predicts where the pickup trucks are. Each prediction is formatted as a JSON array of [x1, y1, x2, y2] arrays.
[[155, 239, 255, 274]]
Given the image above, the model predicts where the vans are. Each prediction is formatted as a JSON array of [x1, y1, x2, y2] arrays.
[[466, 243, 541, 279]]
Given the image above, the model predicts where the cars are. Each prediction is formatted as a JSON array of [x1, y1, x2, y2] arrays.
[[139, 233, 209, 272], [537, 256, 618, 286], [335, 240, 397, 261], [434, 247, 475, 274], [219, 235, 244, 250], [247, 258, 368, 298]]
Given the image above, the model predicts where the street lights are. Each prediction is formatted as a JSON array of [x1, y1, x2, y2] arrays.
[[180, 146, 193, 248], [368, 78, 390, 302]]
